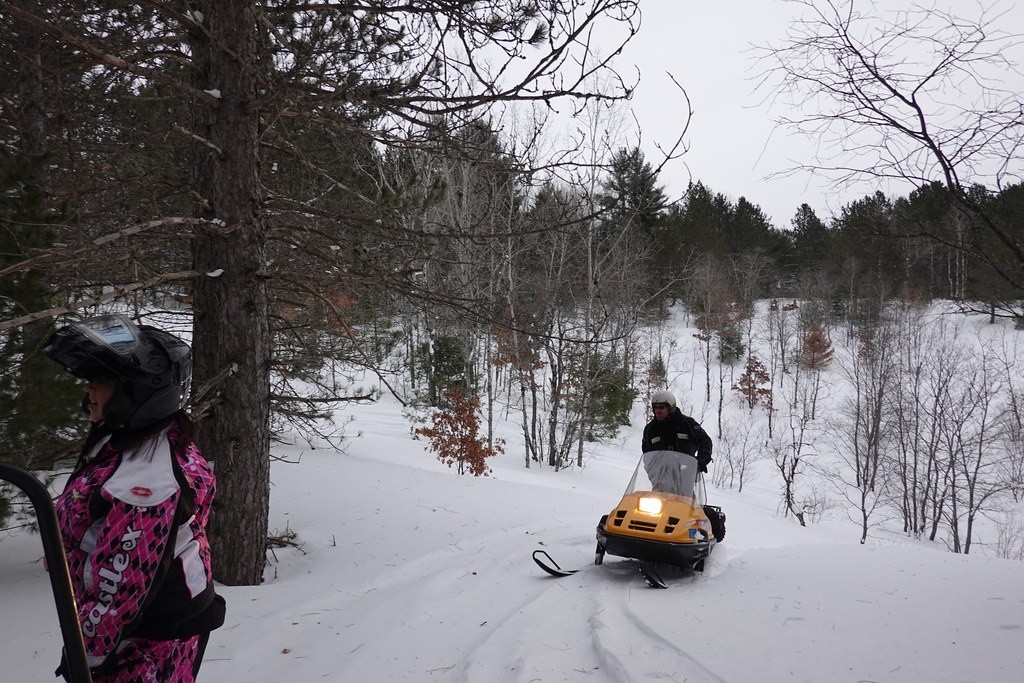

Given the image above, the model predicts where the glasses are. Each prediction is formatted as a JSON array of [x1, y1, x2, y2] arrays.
[[654, 405, 670, 409]]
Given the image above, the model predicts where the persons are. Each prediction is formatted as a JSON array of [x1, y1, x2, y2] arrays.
[[642, 390, 712, 499], [39, 314, 226, 683]]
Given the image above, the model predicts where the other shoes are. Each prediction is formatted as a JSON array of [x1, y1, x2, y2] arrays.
[[715, 512, 726, 542]]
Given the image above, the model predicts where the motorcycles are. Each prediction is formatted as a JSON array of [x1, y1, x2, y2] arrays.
[[782, 303, 799, 311], [595, 452, 730, 587], [770, 303, 779, 311]]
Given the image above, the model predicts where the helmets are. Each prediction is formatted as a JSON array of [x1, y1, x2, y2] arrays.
[[652, 391, 676, 412], [43, 314, 193, 440]]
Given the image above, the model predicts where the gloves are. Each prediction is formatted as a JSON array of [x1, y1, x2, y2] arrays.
[[55, 647, 99, 683]]
